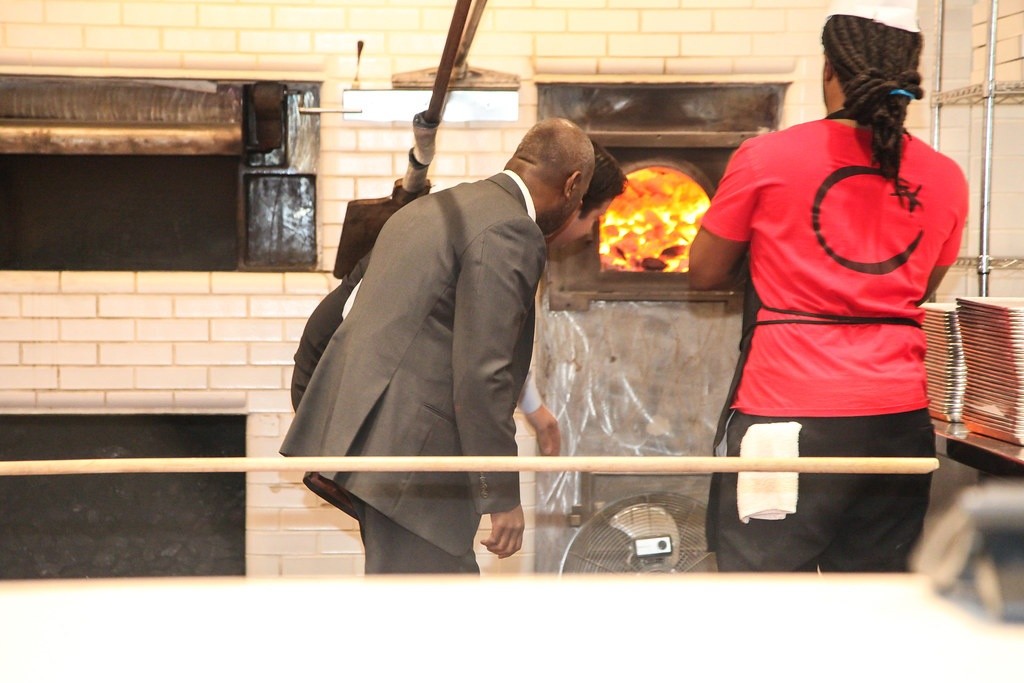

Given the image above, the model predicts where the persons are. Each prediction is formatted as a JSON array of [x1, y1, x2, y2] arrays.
[[688, 0, 969, 573], [280, 117, 629, 575]]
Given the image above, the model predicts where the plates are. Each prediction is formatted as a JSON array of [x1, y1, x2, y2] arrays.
[[918, 296, 1024, 447]]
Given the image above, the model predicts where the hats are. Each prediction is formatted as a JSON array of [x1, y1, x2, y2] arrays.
[[827, 0, 921, 33]]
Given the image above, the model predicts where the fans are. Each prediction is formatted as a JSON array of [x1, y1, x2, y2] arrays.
[[558, 491, 720, 573]]
